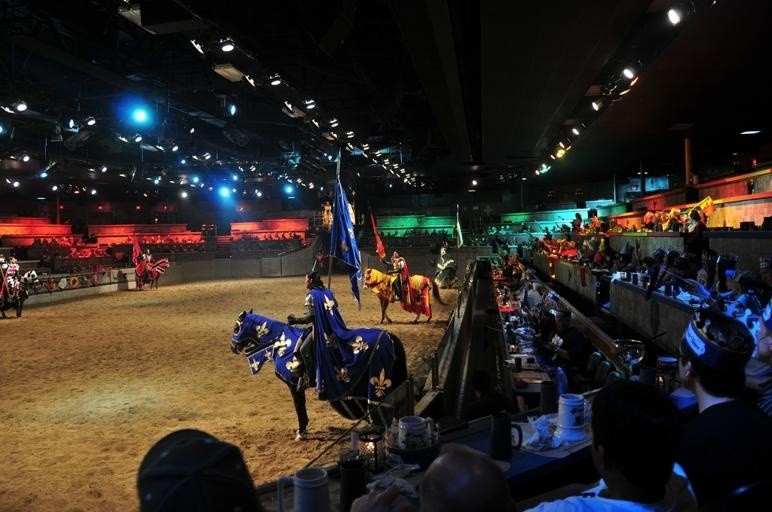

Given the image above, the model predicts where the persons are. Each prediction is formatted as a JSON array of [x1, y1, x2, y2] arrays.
[[2, 256, 22, 303], [351, 442, 519, 512], [286, 271, 347, 402], [139, 246, 157, 280], [380, 248, 408, 302], [18, 235, 125, 280]]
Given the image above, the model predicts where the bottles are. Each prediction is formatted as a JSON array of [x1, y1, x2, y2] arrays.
[[555, 393, 587, 442], [502, 289, 507, 305]]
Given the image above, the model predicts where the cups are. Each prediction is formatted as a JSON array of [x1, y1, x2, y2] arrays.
[[631, 274, 679, 296], [640, 367, 649, 384], [398, 414, 434, 446], [491, 411, 522, 462], [278, 468, 330, 511], [612, 338, 643, 367], [515, 357, 521, 372], [655, 358, 678, 393]]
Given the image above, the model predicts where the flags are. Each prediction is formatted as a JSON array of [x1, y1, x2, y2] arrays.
[[331, 174, 362, 310], [369, 209, 385, 260], [456, 203, 463, 248], [127, 232, 144, 266]]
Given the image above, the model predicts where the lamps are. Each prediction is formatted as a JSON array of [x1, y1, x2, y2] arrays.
[[2, 100, 28, 117], [116, 130, 143, 145], [667, 7, 689, 28], [156, 142, 319, 189], [534, 66, 641, 178], [192, 35, 421, 185], [4, 151, 162, 186], [69, 115, 98, 128]]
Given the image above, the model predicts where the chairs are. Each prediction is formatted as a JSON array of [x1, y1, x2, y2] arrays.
[[26, 238, 305, 272]]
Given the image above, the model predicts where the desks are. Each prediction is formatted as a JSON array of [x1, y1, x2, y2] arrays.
[[257, 409, 598, 512], [489, 224, 772, 410]]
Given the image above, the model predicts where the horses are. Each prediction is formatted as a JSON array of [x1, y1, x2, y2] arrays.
[[362, 267, 449, 325], [0, 268, 41, 319], [230, 308, 407, 442], [135, 258, 170, 290]]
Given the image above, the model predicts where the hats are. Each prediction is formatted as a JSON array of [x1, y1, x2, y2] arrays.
[[761, 296, 771, 333], [555, 301, 571, 318]]
